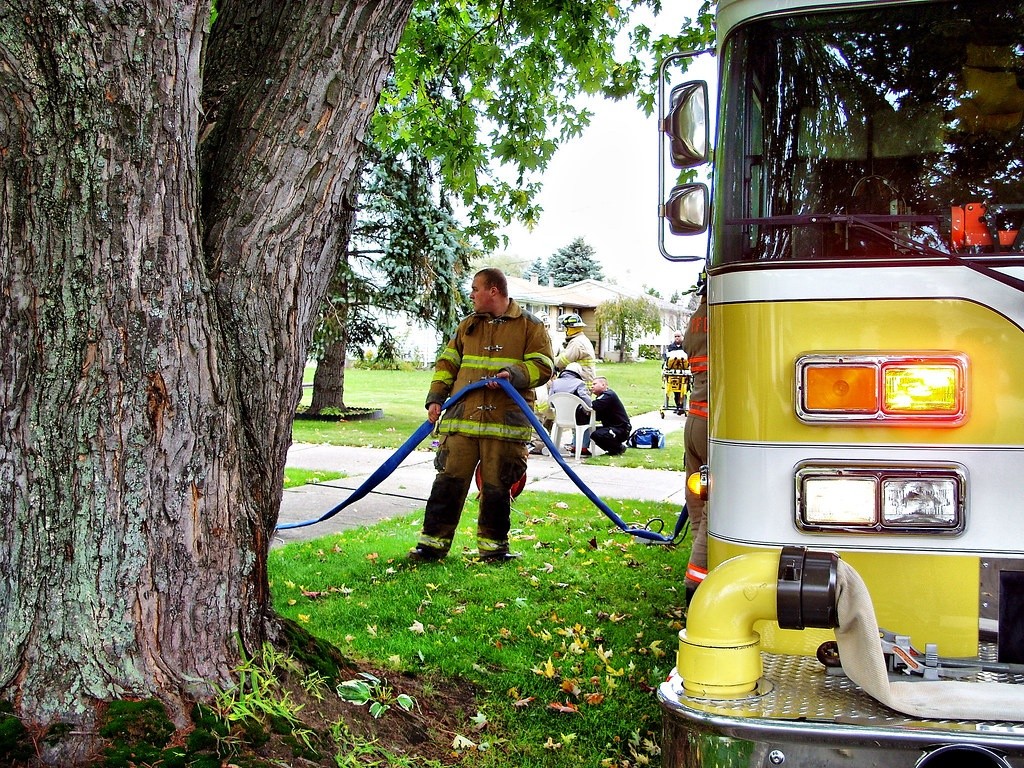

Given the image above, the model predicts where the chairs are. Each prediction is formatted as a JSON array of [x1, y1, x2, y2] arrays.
[[548, 392, 596, 460]]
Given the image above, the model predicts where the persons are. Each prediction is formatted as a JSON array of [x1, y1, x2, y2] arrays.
[[591, 376, 632, 456], [534, 310, 551, 332], [407, 268, 554, 566], [526, 371, 558, 455], [662, 331, 688, 416], [554, 314, 595, 451], [549, 363, 592, 458], [683, 265, 708, 617]]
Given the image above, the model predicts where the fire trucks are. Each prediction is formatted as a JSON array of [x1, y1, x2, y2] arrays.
[[656, 0, 1024, 768]]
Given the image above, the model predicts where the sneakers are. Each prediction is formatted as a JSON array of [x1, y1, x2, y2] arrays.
[[570, 449, 592, 457], [606, 445, 626, 456]]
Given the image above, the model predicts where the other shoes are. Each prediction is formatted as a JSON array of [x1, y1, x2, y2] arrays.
[[674, 407, 685, 414], [686, 587, 698, 606], [564, 444, 573, 450], [478, 552, 518, 563], [409, 545, 447, 562]]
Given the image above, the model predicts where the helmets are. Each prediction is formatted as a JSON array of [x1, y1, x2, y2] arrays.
[[696, 264, 707, 296], [534, 311, 552, 328], [558, 313, 587, 336]]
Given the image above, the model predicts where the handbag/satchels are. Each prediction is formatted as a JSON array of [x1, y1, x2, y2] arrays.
[[627, 428, 666, 449]]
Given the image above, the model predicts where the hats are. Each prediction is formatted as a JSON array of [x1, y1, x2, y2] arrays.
[[565, 362, 582, 375]]
